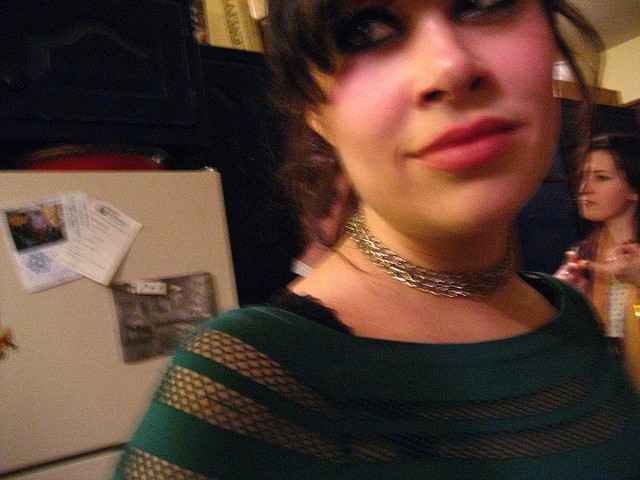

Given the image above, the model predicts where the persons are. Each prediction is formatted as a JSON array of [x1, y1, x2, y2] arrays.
[[550, 128, 639, 393], [111, 0, 640, 480]]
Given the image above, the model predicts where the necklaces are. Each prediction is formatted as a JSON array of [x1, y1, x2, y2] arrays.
[[344, 209, 517, 303]]
